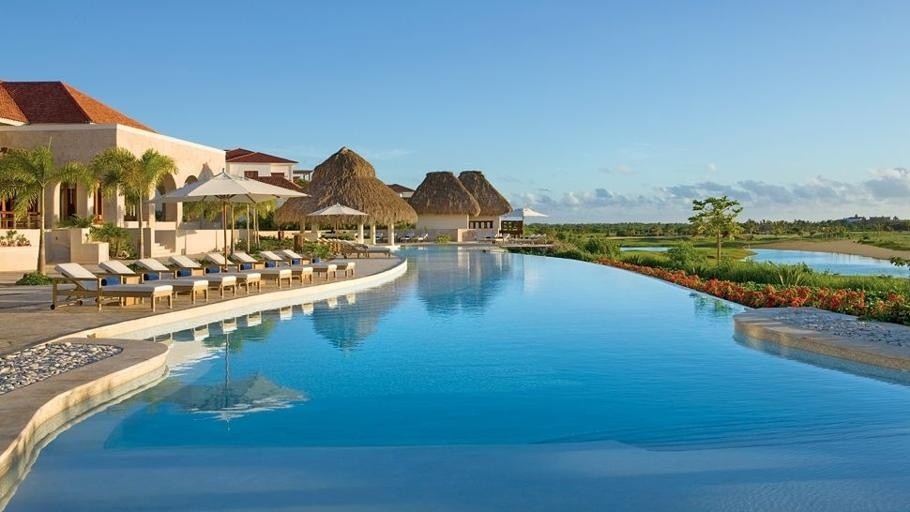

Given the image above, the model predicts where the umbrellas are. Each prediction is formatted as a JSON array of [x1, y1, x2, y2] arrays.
[[503, 207, 547, 238], [307, 200, 368, 253], [145, 167, 312, 271], [136, 333, 312, 424]]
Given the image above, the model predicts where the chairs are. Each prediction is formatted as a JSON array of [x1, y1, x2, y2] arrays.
[[477, 229, 546, 244], [96, 259, 210, 306], [305, 235, 395, 259], [50, 262, 173, 311], [169, 253, 263, 294], [152, 292, 357, 351], [133, 257, 240, 299], [208, 247, 358, 291]]
